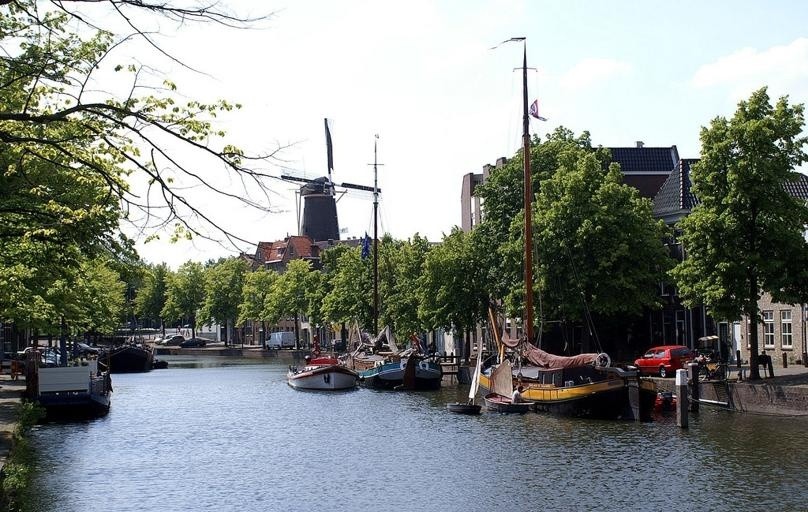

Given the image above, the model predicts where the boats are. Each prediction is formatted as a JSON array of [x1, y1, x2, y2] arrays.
[[286, 353, 360, 391], [483, 390, 536, 414], [446, 401, 483, 417], [40, 354, 112, 419], [108, 339, 156, 374]]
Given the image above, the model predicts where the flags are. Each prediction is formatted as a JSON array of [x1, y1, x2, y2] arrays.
[[529, 100, 549, 121]]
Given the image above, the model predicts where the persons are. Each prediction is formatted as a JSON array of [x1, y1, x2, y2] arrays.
[[510, 385, 534, 404]]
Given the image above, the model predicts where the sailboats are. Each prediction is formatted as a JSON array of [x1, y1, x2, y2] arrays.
[[352, 131, 445, 392], [471, 35, 661, 421]]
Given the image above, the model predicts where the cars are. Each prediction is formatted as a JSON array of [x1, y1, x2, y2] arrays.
[[632, 344, 694, 378], [154, 334, 207, 348]]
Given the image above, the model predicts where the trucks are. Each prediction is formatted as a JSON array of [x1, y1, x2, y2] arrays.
[[265, 331, 295, 350]]
